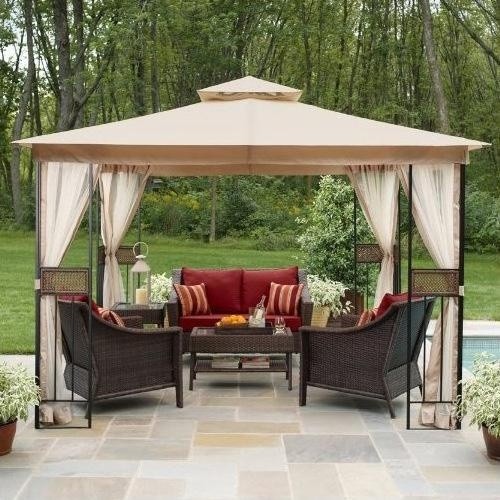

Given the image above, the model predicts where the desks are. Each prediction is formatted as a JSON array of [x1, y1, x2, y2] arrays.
[[111, 302, 166, 329]]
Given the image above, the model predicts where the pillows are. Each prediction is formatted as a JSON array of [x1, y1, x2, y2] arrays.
[[357, 308, 378, 327], [173, 282, 212, 316], [59, 293, 99, 316], [375, 291, 422, 320], [266, 281, 305, 316], [97, 306, 125, 327]]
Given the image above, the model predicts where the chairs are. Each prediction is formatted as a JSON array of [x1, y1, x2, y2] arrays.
[[57, 299, 183, 421], [296, 296, 438, 421]]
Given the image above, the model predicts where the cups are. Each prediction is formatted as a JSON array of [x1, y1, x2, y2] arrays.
[[274, 316, 285, 330], [142, 324, 158, 328], [249, 307, 266, 328]]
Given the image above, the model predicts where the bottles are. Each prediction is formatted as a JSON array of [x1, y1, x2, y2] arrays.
[[253, 295, 266, 318]]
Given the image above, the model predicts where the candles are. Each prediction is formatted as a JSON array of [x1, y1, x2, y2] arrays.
[[136, 288, 147, 303]]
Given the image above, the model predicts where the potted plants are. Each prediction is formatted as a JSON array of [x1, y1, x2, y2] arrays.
[[305, 273, 356, 327], [452, 351, 500, 462], [1, 360, 44, 457], [292, 172, 378, 322], [143, 272, 173, 328]]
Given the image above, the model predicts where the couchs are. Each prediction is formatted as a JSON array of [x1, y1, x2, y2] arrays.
[[167, 265, 314, 354]]
